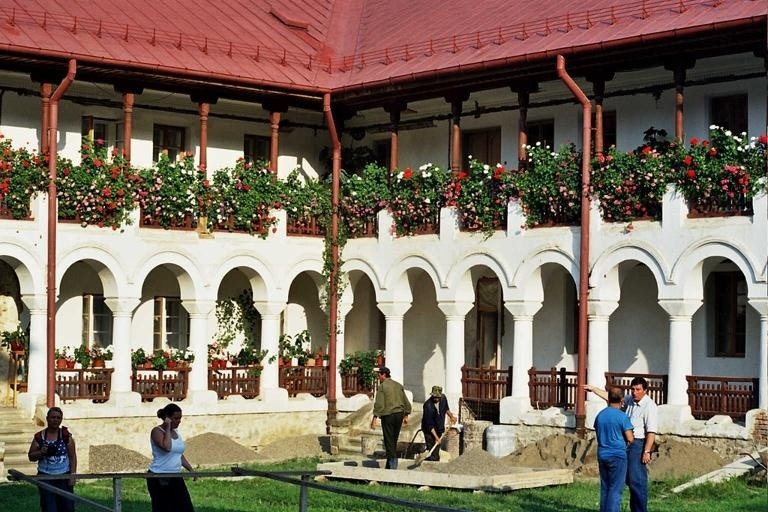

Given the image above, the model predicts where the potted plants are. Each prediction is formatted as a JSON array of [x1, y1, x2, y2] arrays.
[[1, 325, 25, 352], [278, 328, 329, 367], [131, 347, 195, 370]]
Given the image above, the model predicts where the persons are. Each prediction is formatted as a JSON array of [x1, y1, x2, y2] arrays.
[[593, 388, 634, 511], [28, 407, 77, 512], [371, 368, 411, 469], [146, 404, 198, 512], [580, 377, 658, 512], [421, 386, 457, 461]]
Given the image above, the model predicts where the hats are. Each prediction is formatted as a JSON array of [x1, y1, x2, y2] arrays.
[[430, 386, 442, 397]]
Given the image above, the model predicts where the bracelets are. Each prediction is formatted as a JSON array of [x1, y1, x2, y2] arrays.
[[643, 451, 650, 453]]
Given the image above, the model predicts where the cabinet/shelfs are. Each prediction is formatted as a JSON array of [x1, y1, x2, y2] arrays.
[[4, 349, 29, 407]]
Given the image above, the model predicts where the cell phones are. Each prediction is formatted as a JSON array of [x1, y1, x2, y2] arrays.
[[161, 416, 170, 421]]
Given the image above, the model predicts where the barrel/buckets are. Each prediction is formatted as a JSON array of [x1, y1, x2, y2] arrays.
[[462, 420, 493, 452], [487, 425, 516, 457]]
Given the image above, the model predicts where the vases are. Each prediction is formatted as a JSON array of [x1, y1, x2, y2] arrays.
[[376, 354, 386, 368], [55, 344, 114, 369], [211, 353, 228, 368]]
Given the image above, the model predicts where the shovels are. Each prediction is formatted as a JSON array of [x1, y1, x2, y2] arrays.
[[407, 421, 455, 470]]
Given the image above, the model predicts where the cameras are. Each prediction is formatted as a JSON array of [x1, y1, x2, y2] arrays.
[[48, 445, 56, 455]]
